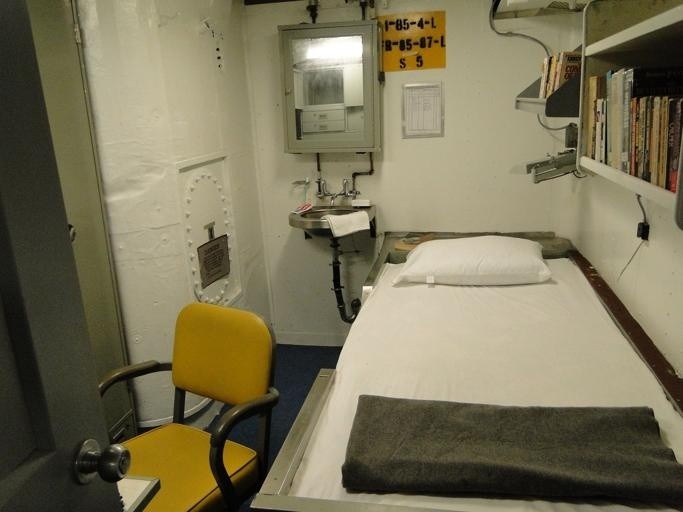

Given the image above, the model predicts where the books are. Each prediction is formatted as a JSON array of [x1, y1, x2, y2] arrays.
[[585, 67, 683, 193], [538, 51, 582, 99]]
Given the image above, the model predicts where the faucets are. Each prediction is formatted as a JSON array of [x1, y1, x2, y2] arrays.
[[330, 192, 345, 207]]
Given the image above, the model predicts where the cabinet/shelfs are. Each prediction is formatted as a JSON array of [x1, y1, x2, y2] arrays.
[[576, 1, 682, 232], [300, 103, 348, 132]]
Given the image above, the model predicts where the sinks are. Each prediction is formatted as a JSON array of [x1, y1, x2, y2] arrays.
[[289, 205, 377, 236]]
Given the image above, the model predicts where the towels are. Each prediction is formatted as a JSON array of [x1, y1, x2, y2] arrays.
[[320, 209, 371, 238]]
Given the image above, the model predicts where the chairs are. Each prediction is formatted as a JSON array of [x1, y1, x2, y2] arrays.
[[97, 301, 281, 511]]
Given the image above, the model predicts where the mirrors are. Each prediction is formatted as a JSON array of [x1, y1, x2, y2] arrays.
[[276, 19, 382, 153]]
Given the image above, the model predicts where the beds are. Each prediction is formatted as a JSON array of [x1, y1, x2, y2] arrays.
[[251, 231, 683, 511]]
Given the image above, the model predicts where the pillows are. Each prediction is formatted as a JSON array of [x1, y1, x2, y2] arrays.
[[392, 235, 554, 286]]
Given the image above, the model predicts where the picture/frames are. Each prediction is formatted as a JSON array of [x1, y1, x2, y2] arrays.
[[401, 82, 445, 139]]
